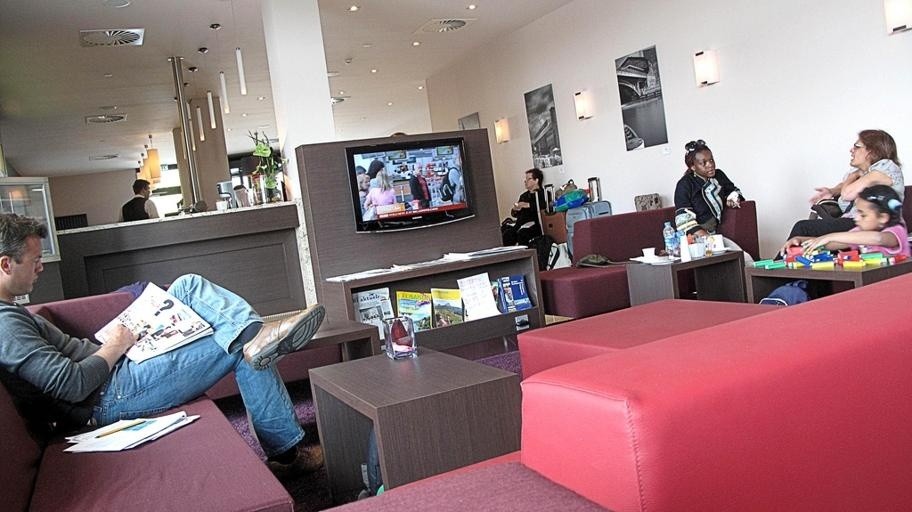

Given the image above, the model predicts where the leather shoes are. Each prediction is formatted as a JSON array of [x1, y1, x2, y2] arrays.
[[261, 442, 326, 482], [241, 303, 328, 374]]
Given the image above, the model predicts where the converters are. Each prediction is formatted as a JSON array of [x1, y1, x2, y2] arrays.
[[516, 320, 530, 326]]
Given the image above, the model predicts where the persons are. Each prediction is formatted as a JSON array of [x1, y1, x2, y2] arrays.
[[772, 128, 908, 261], [354, 150, 467, 223], [673, 138, 745, 238], [116, 178, 161, 221], [780, 183, 910, 257], [0, 210, 327, 487], [501, 169, 547, 249]]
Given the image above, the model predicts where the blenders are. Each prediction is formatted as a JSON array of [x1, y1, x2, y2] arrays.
[[216, 181, 237, 211]]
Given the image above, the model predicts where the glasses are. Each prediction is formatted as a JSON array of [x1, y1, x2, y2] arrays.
[[853, 143, 867, 150]]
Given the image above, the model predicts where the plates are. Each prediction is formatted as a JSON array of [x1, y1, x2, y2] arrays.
[[707, 247, 729, 256]]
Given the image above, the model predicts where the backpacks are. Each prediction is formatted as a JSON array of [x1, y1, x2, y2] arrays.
[[757, 278, 811, 308], [437, 166, 461, 202]]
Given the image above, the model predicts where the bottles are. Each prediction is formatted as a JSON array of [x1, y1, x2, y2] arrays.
[[661, 222, 713, 262]]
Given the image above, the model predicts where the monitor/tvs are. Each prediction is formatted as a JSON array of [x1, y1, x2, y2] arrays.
[[344, 136, 476, 234]]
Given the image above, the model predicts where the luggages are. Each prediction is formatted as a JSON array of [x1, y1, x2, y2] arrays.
[[565, 176, 613, 266], [539, 183, 567, 246]]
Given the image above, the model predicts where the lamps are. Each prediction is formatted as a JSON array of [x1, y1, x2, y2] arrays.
[[494, 115, 510, 145], [690, 48, 719, 89], [571, 88, 596, 122], [881, 0, 912, 38], [137, 0, 248, 194]]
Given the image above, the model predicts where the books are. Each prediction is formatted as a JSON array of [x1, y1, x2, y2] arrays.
[[94, 281, 217, 367], [64, 412, 204, 455], [329, 241, 535, 345]]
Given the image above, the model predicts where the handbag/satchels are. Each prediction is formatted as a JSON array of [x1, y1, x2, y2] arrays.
[[574, 253, 611, 269], [552, 178, 592, 213], [526, 233, 573, 272]]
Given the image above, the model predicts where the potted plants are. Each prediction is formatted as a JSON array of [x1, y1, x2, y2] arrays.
[[246, 129, 290, 204]]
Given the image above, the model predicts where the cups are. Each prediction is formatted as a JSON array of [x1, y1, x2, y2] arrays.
[[642, 247, 656, 259], [710, 234, 725, 250], [383, 316, 418, 360]]
